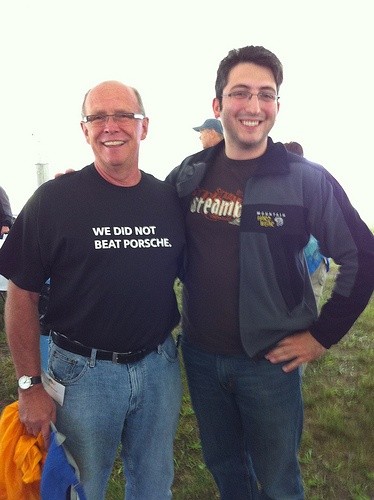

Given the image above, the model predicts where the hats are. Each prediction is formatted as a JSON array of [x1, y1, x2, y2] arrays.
[[192, 119, 223, 134]]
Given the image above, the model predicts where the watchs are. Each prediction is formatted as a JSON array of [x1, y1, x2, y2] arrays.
[[15, 374, 41, 390]]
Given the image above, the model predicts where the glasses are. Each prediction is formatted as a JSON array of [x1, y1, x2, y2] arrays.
[[83, 113, 145, 122], [221, 90, 280, 102]]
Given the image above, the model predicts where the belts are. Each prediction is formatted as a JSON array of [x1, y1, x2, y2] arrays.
[[51, 330, 156, 364]]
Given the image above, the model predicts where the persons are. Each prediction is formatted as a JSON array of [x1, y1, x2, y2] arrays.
[[284, 141, 329, 377], [0, 81, 185, 500], [192, 118, 225, 152], [0, 183, 12, 239], [54, 45, 374, 499]]
